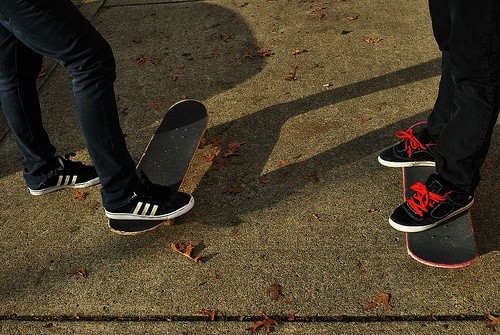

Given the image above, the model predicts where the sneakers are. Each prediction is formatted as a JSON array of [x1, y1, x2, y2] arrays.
[[27, 152, 101, 196], [100, 181, 195, 220], [388, 173, 475, 233], [378, 129, 436, 168]]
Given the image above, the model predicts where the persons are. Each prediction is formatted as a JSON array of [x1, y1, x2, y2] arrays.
[[0, 0, 195, 220], [377, 0, 500, 232]]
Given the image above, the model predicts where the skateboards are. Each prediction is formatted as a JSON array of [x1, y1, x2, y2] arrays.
[[402, 119, 479, 269], [107, 98, 210, 236]]
